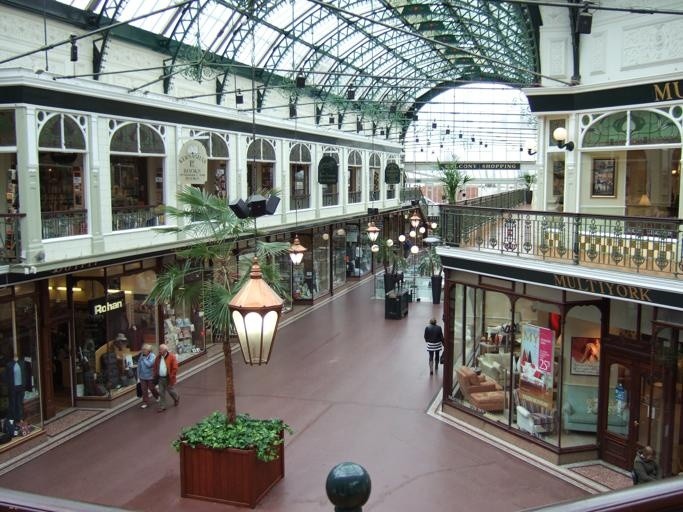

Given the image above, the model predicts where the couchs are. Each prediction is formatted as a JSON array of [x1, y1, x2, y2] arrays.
[[562, 385, 630, 439]]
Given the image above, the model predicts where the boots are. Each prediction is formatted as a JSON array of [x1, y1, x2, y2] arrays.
[[435, 361, 438, 369], [430, 360, 433, 375]]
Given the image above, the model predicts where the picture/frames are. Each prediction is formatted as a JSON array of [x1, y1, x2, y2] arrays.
[[589, 155, 617, 199]]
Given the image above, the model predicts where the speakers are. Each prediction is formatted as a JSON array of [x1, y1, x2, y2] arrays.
[[230, 192, 279, 218]]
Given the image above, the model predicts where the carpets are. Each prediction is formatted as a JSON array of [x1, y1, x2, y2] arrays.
[[567, 464, 633, 491]]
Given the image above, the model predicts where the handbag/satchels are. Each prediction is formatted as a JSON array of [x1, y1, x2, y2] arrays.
[[137, 383, 142, 397], [152, 378, 158, 385], [440, 353, 444, 364]]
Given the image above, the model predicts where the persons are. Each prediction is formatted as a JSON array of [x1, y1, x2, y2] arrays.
[[402, 235, 410, 259], [127, 324, 144, 351], [4, 356, 26, 421], [136, 342, 160, 408], [382, 256, 390, 274], [632, 446, 660, 483], [152, 343, 181, 413], [423, 317, 444, 376], [82, 339, 97, 396]]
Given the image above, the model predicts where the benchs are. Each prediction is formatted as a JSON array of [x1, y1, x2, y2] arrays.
[[468, 391, 508, 412]]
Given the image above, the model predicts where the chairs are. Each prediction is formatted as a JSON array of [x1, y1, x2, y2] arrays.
[[476, 352, 515, 390], [513, 387, 572, 441], [455, 366, 502, 400]]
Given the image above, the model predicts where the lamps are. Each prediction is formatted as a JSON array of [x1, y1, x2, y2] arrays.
[[226, 0, 283, 368], [524, 139, 537, 155], [279, 0, 423, 266], [552, 126, 574, 151]]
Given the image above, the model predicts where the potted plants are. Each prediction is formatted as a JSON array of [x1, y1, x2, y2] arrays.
[[520, 173, 536, 204], [431, 152, 474, 247], [366, 227, 443, 320], [140, 177, 297, 511]]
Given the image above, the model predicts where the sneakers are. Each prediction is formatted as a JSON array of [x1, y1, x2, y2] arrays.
[[140, 397, 179, 413]]
